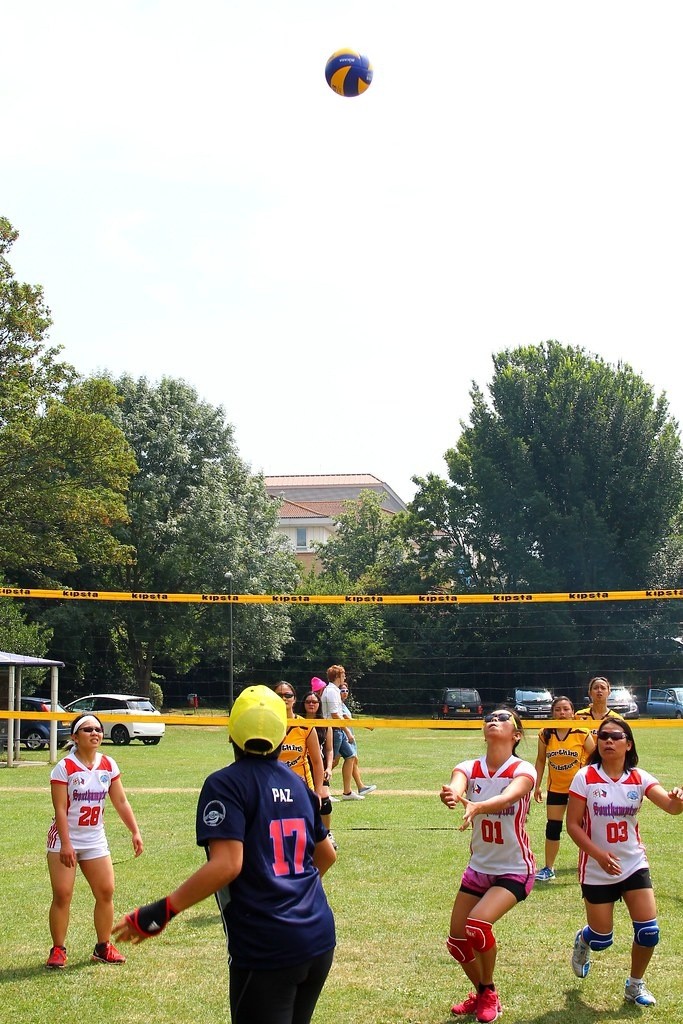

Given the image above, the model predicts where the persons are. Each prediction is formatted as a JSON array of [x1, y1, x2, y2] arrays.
[[271, 681, 324, 797], [46, 714, 144, 969], [574, 677, 625, 746], [111, 686, 338, 1024], [439, 707, 537, 1024], [310, 665, 377, 802], [298, 691, 338, 851], [535, 696, 596, 881], [566, 718, 683, 1007]]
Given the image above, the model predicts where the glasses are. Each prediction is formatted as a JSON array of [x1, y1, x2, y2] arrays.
[[304, 700, 320, 704], [598, 731, 627, 741], [79, 727, 102, 733], [484, 713, 518, 730], [278, 693, 294, 698], [340, 689, 349, 693]]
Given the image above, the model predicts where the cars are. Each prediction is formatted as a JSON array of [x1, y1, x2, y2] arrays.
[[645, 688, 683, 720], [583, 686, 640, 721]]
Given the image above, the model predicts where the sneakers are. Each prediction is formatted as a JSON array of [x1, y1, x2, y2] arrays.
[[343, 791, 365, 801], [358, 785, 376, 796], [450, 990, 503, 1017], [46, 945, 67, 969], [535, 867, 556, 881], [476, 986, 499, 1023], [91, 940, 126, 966], [624, 979, 657, 1008], [571, 929, 591, 978]]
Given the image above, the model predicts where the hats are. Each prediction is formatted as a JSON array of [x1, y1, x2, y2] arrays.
[[227, 684, 287, 755], [311, 677, 326, 692]]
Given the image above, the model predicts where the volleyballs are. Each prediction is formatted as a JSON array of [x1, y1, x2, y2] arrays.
[[325, 48, 373, 98]]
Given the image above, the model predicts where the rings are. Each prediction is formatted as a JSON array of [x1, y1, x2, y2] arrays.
[[129, 934, 135, 940]]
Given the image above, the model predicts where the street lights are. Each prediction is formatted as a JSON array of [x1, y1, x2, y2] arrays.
[[224, 570, 235, 716]]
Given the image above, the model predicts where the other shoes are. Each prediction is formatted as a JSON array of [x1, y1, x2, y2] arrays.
[[327, 832, 338, 851], [329, 796, 340, 802]]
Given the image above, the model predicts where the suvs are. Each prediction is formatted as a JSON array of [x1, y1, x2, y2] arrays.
[[62, 694, 166, 746], [429, 686, 485, 720], [0, 697, 75, 751], [501, 687, 560, 720]]
